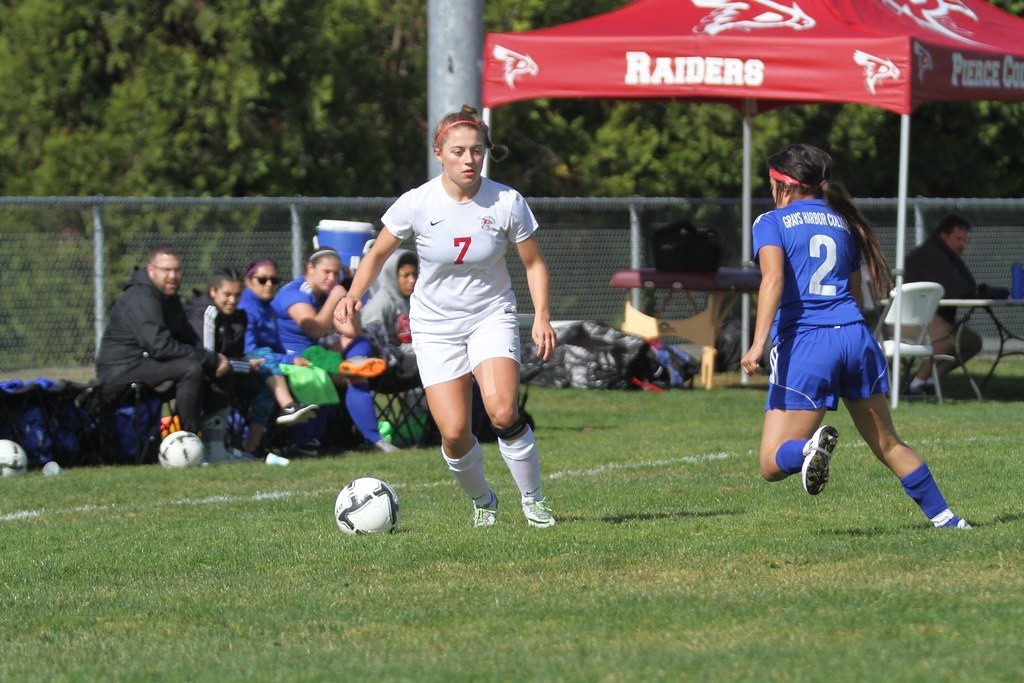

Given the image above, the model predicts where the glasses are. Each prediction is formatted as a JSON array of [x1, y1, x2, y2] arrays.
[[251, 275, 281, 285]]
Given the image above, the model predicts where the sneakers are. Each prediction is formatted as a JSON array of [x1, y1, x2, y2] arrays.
[[473, 490, 499, 528], [521, 496, 557, 526], [802, 425, 839, 496], [939, 517, 970, 530]]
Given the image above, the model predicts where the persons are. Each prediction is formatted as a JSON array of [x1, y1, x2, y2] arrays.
[[333, 106, 558, 530], [902, 216, 1005, 394], [739, 144, 973, 530], [97, 243, 418, 465]]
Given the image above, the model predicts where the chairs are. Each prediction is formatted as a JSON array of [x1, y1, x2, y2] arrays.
[[858, 263, 944, 410]]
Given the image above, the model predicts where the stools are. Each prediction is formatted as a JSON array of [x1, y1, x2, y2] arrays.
[[1, 355, 529, 470]]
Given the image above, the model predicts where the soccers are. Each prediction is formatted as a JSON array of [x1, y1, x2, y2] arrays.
[[334, 476, 400, 536], [158, 430, 205, 470], [1, 438, 28, 478]]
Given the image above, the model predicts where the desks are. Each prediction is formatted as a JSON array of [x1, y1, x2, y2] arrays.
[[878, 299, 1024, 400], [610, 267, 762, 391]]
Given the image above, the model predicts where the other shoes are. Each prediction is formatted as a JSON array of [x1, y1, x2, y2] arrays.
[[277, 403, 320, 426], [208, 452, 237, 463], [366, 440, 398, 452], [906, 384, 932, 401], [340, 356, 387, 378]]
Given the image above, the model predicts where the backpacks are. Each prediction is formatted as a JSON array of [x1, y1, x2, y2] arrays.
[[154, 266, 182, 274], [0, 377, 160, 469], [631, 340, 700, 391]]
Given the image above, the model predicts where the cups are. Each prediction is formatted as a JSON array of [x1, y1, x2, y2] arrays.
[[266, 452, 290, 468]]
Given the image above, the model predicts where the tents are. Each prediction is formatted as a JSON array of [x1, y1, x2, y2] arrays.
[[483, 0, 1024, 413]]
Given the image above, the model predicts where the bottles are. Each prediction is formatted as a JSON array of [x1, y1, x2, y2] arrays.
[[1011, 261, 1024, 299]]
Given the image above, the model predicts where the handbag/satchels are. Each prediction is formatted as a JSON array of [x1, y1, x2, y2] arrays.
[[647, 222, 722, 273]]
